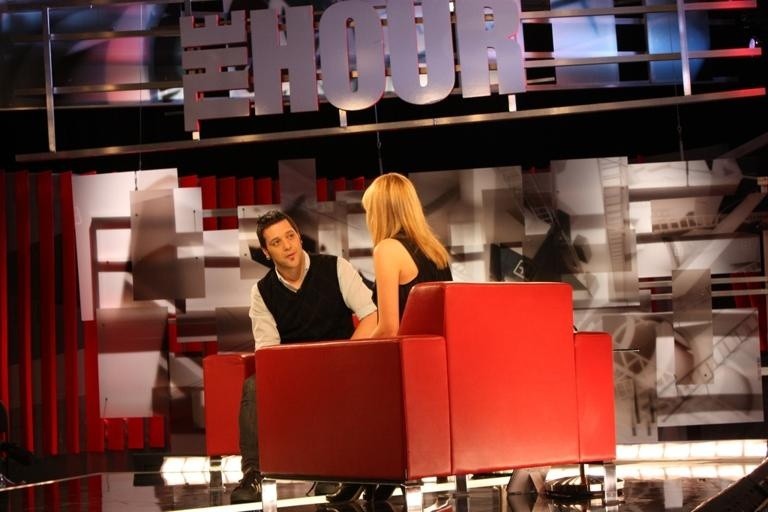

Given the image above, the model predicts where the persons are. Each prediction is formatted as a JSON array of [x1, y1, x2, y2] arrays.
[[229, 209, 380, 505], [316, 502, 409, 512], [324, 169, 452, 508]]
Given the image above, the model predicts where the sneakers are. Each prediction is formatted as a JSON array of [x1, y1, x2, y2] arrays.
[[230, 471, 262, 503], [314, 483, 339, 494]]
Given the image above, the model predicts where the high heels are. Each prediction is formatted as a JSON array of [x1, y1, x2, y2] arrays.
[[362, 485, 405, 502], [325, 484, 376, 503]]
